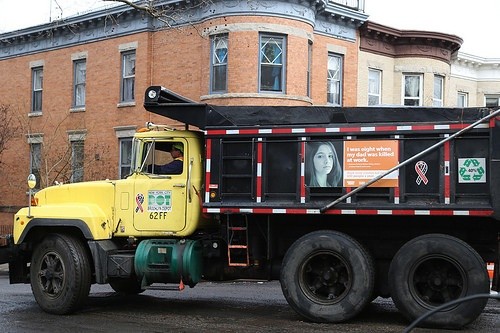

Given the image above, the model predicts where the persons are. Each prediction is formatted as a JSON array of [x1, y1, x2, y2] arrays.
[[303, 142, 342, 186], [146, 143, 183, 177]]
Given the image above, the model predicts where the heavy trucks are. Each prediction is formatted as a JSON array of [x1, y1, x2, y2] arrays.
[[7, 86, 500, 329]]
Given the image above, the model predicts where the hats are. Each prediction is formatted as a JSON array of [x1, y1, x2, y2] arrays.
[[172, 143, 184, 152]]
